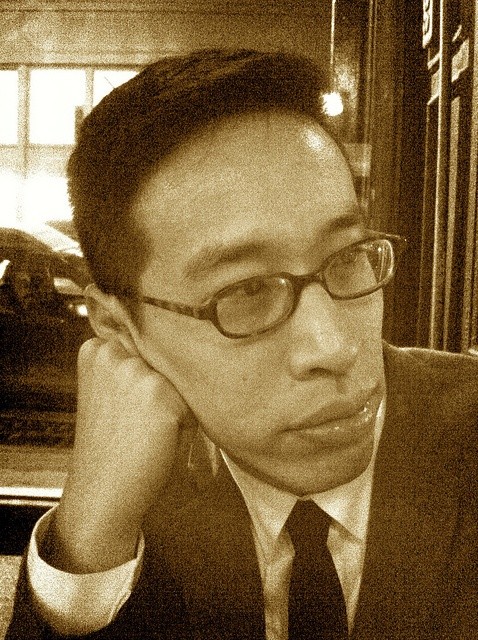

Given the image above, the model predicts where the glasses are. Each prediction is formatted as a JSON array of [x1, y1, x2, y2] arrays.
[[98, 229, 408, 338]]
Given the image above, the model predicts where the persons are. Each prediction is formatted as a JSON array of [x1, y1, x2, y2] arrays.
[[8, 48, 477, 640]]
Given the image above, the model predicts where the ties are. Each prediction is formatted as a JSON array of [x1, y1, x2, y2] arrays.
[[287, 499, 348, 640]]
